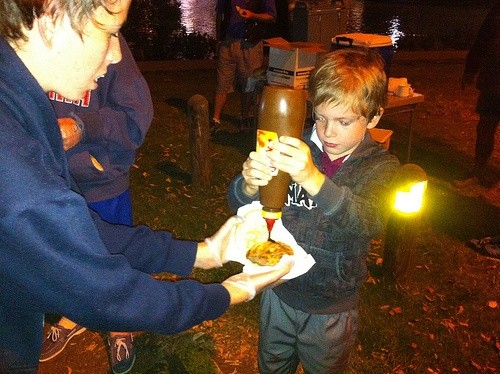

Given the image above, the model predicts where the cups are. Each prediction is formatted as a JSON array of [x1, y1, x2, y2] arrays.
[[398, 83, 411, 97]]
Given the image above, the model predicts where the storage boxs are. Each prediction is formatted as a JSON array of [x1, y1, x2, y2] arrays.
[[260, 36, 328, 89], [331, 32, 394, 92]]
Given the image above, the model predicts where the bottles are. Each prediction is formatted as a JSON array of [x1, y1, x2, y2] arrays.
[[254, 85, 308, 233]]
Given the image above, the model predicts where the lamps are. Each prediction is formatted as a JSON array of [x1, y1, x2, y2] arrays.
[[389, 162, 429, 217]]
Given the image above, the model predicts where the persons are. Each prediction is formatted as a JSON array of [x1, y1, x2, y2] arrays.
[[39, 28, 154, 374], [227, 46, 401, 374], [208, 0, 276, 130], [0, 0, 292, 374], [462, 0, 500, 167]]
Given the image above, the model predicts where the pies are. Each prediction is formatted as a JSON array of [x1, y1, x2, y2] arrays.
[[247, 240, 294, 266]]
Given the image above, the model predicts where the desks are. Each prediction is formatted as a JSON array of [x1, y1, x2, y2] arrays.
[[247, 78, 425, 164]]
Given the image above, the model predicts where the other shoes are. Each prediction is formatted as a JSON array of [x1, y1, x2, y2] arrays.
[[209, 120, 223, 139], [240, 117, 251, 133], [472, 156, 488, 185]]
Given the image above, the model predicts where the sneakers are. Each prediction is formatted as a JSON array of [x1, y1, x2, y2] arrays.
[[106, 331, 136, 374], [39, 324, 86, 362]]
